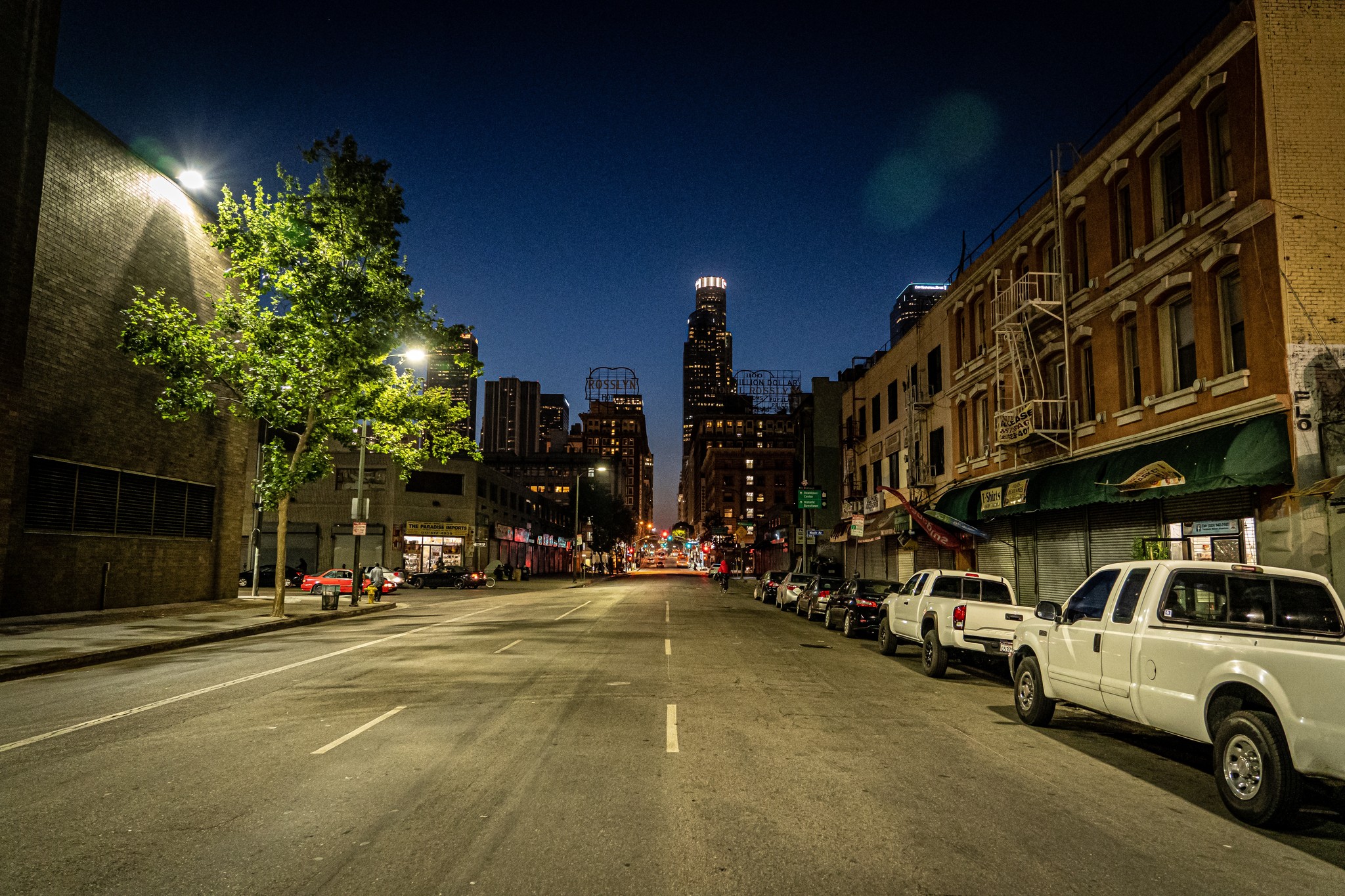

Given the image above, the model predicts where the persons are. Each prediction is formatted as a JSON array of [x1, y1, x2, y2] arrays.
[[343, 571, 352, 579], [295, 558, 307, 575], [435, 557, 451, 573], [370, 562, 385, 602], [394, 567, 406, 579], [635, 550, 647, 558], [810, 555, 839, 579], [695, 557, 702, 567], [693, 562, 698, 571], [718, 560, 732, 591], [622, 561, 630, 571], [580, 561, 609, 574], [493, 564, 530, 581], [1165, 591, 1186, 618], [358, 563, 366, 602]]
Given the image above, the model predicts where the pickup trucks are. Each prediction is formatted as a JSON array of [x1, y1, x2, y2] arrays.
[[878, 567, 1036, 679], [1007, 559, 1345, 835]]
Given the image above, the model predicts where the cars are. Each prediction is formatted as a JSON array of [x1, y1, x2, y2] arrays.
[[238, 564, 488, 595], [753, 570, 906, 639], [646, 549, 692, 569], [708, 563, 721, 578], [584, 563, 592, 571]]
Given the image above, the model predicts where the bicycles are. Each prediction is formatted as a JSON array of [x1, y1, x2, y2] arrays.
[[748, 567, 754, 574], [486, 577, 495, 588], [718, 572, 730, 594]]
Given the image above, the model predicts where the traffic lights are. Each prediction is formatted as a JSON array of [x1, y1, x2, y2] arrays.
[[733, 533, 737, 541], [628, 548, 634, 561], [686, 543, 690, 549], [704, 556, 707, 560], [644, 543, 647, 548], [663, 530, 672, 541], [704, 546, 708, 554]]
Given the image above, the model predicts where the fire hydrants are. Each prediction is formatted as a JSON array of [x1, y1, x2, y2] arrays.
[[364, 585, 378, 604]]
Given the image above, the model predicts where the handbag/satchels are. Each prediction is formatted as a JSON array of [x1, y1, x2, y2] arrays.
[[713, 572, 719, 581]]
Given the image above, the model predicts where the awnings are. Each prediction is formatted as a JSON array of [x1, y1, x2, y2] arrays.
[[830, 524, 851, 542], [934, 414, 1294, 525], [857, 510, 904, 543]]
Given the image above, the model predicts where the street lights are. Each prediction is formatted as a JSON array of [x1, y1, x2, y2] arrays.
[[643, 524, 651, 545], [572, 464, 607, 583], [775, 429, 807, 573], [349, 347, 425, 607], [680, 526, 688, 554]]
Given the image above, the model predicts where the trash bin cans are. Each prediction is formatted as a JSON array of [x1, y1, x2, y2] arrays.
[[321, 584, 340, 610]]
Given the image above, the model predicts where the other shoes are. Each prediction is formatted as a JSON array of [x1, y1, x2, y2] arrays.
[[720, 583, 722, 584], [358, 596, 361, 601]]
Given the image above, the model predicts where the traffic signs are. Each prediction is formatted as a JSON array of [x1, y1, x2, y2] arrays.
[[798, 489, 827, 509], [353, 522, 366, 536], [473, 541, 487, 547]]
